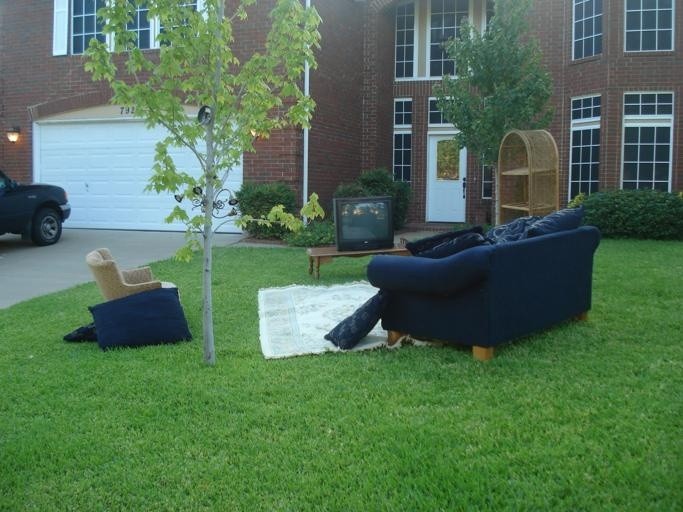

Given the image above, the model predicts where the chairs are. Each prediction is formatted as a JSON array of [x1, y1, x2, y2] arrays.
[[87, 248, 178, 301]]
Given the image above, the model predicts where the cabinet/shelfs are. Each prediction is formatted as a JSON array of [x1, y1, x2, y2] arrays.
[[495, 129, 559, 225]]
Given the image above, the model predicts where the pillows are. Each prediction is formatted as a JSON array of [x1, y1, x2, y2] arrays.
[[88, 288, 192, 352], [406, 204, 581, 257]]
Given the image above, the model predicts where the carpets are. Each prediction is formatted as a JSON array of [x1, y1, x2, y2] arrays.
[[257, 280, 443, 359]]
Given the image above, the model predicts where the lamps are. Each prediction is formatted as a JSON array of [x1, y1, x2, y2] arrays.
[[6, 126, 20, 143]]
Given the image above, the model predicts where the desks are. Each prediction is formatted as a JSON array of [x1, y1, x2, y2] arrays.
[[306, 243, 408, 281]]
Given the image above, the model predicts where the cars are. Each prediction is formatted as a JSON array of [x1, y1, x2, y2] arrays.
[[0, 170, 71, 246]]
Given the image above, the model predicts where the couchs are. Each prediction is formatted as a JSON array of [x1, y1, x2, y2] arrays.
[[367, 225, 601, 362]]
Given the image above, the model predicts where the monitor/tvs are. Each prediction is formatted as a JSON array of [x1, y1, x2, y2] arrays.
[[335, 196, 394, 251]]
